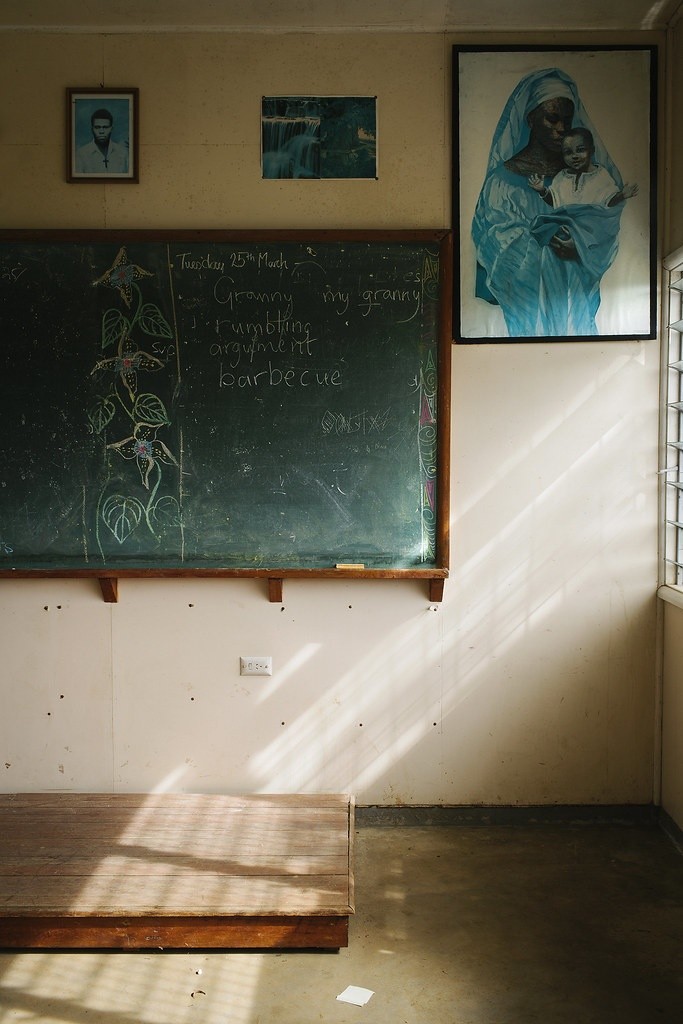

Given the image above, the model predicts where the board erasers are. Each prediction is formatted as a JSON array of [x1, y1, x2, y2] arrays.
[[335, 563, 365, 569]]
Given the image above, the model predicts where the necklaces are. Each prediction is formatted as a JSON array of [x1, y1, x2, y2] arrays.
[[96, 150, 113, 168]]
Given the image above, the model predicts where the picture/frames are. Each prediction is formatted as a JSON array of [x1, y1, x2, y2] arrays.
[[452, 44, 657, 344], [64, 87, 140, 184]]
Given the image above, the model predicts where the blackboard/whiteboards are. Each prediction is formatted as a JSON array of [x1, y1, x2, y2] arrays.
[[0, 225, 454, 581]]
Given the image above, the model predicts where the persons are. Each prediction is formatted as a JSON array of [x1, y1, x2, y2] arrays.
[[76, 108, 129, 173]]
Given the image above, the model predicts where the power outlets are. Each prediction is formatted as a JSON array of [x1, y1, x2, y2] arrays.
[[240, 657, 273, 676]]
